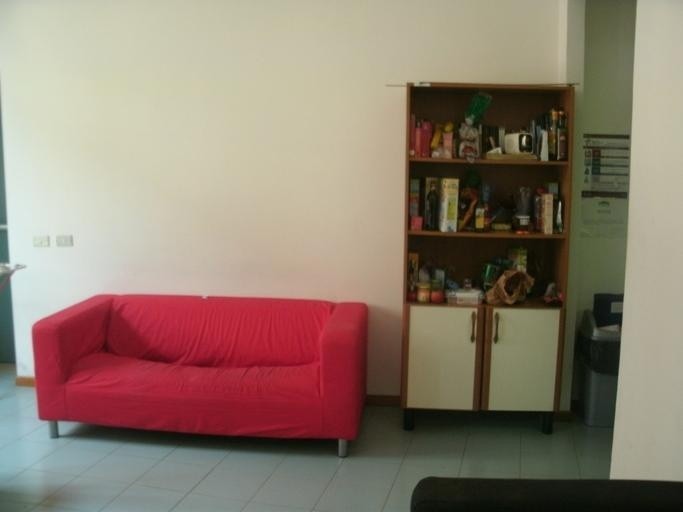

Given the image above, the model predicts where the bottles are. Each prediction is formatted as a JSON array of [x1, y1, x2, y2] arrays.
[[474, 188, 485, 230], [548, 110, 567, 159], [424, 182, 438, 230]]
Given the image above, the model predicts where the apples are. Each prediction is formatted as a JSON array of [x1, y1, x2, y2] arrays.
[[430, 290, 444, 303], [406, 292, 416, 302]]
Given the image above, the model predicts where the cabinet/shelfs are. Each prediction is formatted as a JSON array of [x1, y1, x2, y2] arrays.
[[402, 83, 576, 437]]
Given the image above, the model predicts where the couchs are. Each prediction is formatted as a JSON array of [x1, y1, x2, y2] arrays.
[[31, 292, 370, 459]]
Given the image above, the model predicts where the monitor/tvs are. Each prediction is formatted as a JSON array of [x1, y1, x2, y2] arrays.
[[408, 476, 683, 512]]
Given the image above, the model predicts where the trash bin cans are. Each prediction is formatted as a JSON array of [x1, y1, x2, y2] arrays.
[[576, 310, 623, 427]]
